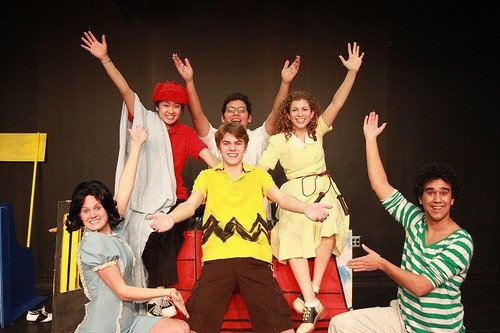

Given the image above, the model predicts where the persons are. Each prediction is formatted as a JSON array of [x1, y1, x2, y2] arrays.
[[328, 111, 475, 333], [77, 28, 220, 318], [169, 51, 302, 228], [66, 122, 191, 333], [257, 40, 365, 333], [144, 122, 333, 333]]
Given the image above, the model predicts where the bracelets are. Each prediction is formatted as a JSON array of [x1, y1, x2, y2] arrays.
[[102, 58, 111, 65]]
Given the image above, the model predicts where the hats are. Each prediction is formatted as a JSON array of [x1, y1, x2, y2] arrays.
[[152, 81, 191, 107]]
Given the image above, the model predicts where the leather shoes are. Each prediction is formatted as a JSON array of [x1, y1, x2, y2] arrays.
[[296, 302, 329, 333], [292, 284, 320, 314]]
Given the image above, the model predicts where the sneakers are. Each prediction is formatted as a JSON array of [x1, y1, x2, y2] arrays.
[[146, 294, 177, 319]]
